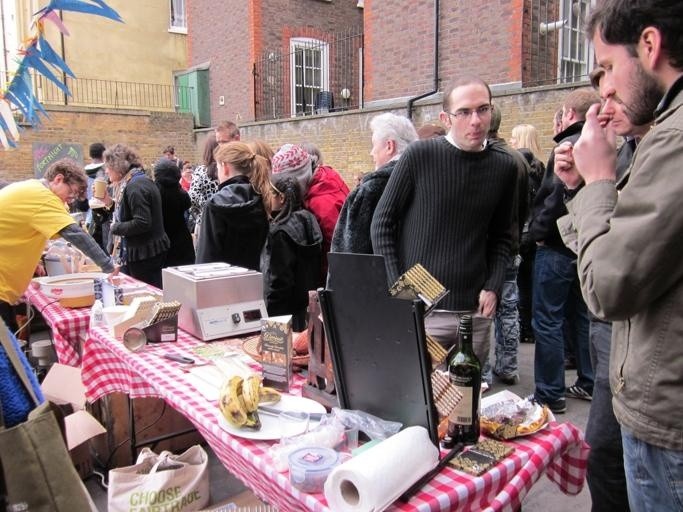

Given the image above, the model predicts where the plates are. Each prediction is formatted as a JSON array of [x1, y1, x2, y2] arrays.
[[482, 399, 548, 436], [217, 396, 326, 441]]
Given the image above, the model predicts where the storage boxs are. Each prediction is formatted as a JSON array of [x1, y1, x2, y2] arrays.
[[38, 361, 108, 485]]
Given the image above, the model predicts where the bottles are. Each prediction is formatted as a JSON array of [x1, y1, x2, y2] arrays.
[[444, 314, 482, 445], [88, 300, 103, 329]]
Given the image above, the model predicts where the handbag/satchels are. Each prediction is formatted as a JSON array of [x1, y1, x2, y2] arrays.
[[107, 443, 211, 512], [0, 313, 97, 512]]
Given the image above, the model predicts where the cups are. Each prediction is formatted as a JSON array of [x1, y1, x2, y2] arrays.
[[331, 416, 360, 457], [94, 180, 106, 198]]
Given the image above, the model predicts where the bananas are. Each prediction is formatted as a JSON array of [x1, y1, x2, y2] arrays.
[[220, 376, 280, 430]]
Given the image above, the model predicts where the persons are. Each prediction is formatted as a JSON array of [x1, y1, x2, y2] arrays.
[[93, 144, 172, 290], [482, 102, 530, 393], [195, 142, 273, 272], [554, 0, 680, 512], [215, 122, 240, 144], [523, 87, 603, 414], [262, 174, 325, 329], [550, 60, 653, 512], [554, 60, 653, 512], [509, 124, 548, 344], [245, 141, 347, 275], [416, 123, 446, 140], [0, 158, 119, 339], [370, 75, 518, 372], [84, 143, 114, 258], [320, 111, 421, 320], [550, 0, 680, 512], [162, 130, 220, 255], [154, 159, 196, 267]]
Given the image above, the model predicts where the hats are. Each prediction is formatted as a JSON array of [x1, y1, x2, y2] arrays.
[[271, 144, 311, 176], [153, 157, 178, 180]]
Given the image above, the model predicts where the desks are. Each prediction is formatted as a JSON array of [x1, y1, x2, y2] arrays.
[[23, 270, 163, 473], [80, 317, 593, 512]]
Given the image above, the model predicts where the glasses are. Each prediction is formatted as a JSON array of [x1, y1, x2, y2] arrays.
[[449, 106, 492, 120]]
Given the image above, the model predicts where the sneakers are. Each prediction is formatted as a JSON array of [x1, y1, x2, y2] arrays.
[[563, 383, 598, 402], [523, 393, 567, 413], [490, 365, 520, 387]]
